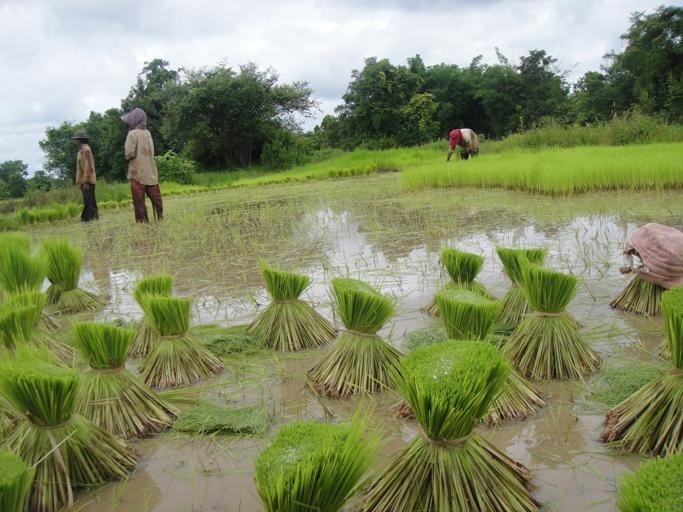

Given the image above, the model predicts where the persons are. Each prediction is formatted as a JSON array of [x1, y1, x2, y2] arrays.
[[71, 132, 100, 220], [447, 125, 478, 160], [120, 107, 163, 222]]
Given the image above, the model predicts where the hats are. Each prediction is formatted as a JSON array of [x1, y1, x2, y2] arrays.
[[73, 129, 90, 139], [121, 108, 146, 129], [449, 128, 461, 146]]
